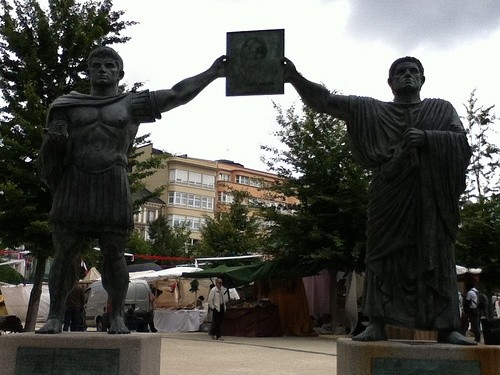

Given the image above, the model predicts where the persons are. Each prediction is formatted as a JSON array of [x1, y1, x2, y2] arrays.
[[208, 278, 226, 340], [190, 295, 204, 310], [457, 277, 500, 342], [280, 56, 480, 346], [35, 47, 229, 334]]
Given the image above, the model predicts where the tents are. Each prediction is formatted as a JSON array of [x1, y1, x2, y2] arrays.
[[129, 266, 217, 331]]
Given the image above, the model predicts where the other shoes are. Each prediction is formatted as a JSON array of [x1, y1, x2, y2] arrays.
[[208, 332, 224, 340]]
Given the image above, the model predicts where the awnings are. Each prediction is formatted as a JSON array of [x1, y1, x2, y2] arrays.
[[181, 260, 274, 286]]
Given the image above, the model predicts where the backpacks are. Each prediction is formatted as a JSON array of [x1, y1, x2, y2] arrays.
[[470, 289, 488, 309]]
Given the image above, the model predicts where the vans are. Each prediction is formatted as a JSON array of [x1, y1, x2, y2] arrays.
[[84, 278, 155, 332]]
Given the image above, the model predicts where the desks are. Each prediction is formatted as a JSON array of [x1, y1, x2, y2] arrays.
[[219, 305, 282, 336], [153, 310, 206, 332]]
